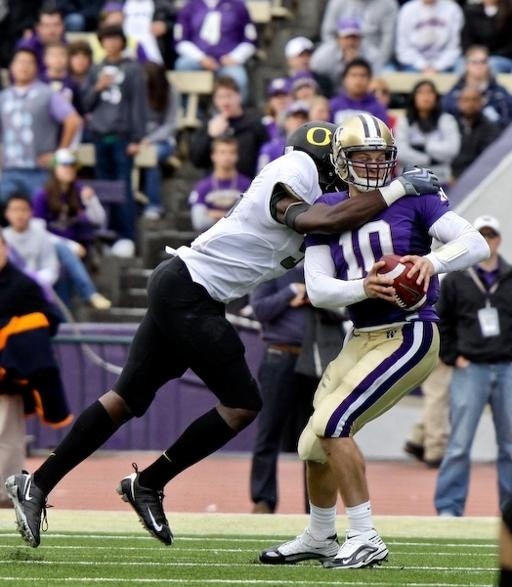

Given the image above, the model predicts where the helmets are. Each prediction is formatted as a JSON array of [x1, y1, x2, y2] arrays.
[[287, 111, 399, 194]]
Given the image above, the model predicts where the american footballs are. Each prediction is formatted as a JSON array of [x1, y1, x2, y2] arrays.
[[377, 255, 427, 312]]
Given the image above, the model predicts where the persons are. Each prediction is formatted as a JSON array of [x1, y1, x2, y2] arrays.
[[243, 256, 344, 516], [400, 356, 456, 470], [256, 111, 494, 570], [4, 117, 453, 550], [0, 0, 511, 328], [0, 233, 74, 506], [432, 215, 511, 518]]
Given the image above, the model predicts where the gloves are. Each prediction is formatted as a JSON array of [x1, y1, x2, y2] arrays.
[[395, 163, 444, 197]]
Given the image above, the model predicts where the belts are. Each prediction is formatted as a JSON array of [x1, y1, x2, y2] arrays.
[[266, 342, 302, 355]]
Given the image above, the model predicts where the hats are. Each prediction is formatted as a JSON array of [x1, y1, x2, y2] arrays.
[[285, 36, 314, 60], [52, 149, 78, 166], [335, 18, 365, 38], [474, 215, 502, 235]]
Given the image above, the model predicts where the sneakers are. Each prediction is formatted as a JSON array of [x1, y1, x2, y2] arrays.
[[325, 526, 390, 568], [5, 468, 48, 550], [83, 290, 115, 313], [257, 530, 339, 566], [117, 464, 173, 547]]
